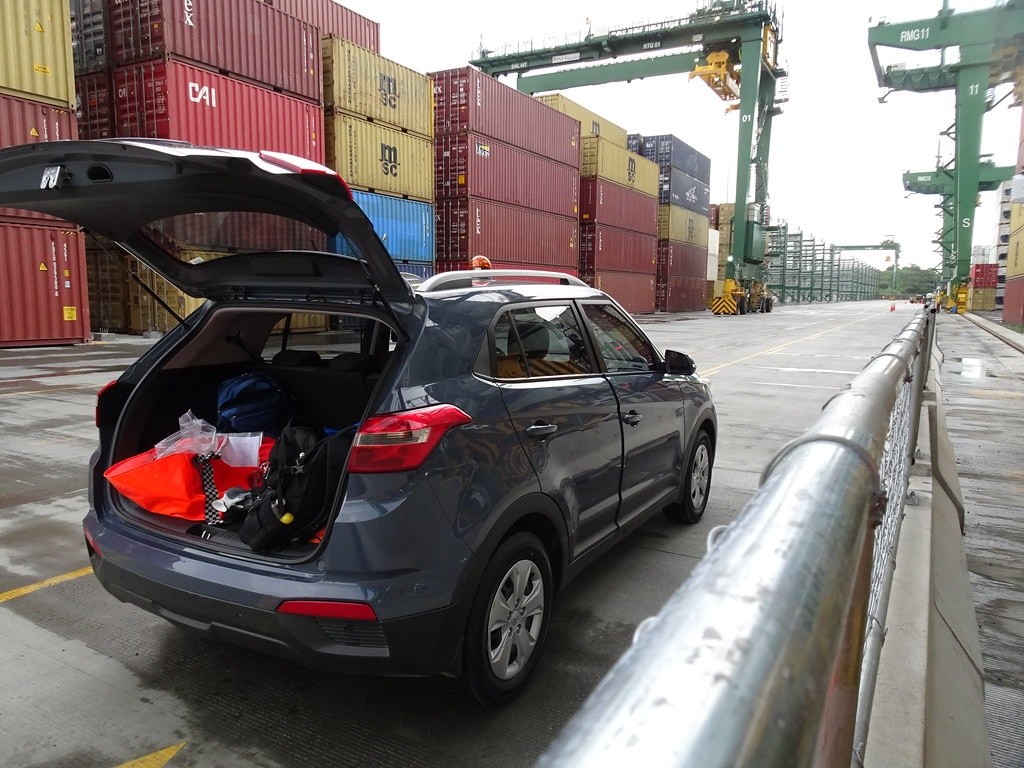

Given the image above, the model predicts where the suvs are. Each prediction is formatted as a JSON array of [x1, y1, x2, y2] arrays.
[[1, 139, 718, 713]]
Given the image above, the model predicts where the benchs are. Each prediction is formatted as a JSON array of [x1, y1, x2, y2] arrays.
[[272, 352, 373, 418]]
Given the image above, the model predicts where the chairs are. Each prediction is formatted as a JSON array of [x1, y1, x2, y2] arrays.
[[505, 324, 549, 359]]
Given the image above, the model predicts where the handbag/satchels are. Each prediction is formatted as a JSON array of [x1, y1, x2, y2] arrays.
[[102, 432, 276, 522]]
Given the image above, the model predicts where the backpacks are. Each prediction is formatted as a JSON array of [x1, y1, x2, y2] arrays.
[[217, 370, 286, 434], [239, 416, 351, 554]]
